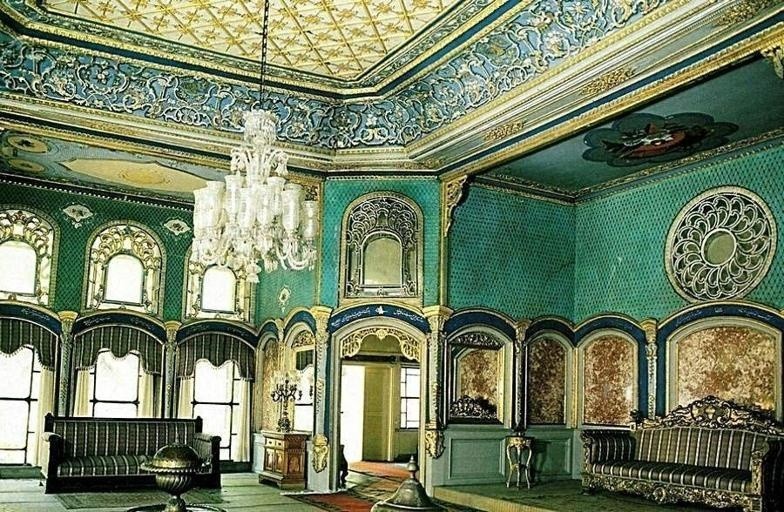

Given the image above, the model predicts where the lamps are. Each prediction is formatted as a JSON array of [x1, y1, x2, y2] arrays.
[[269, 367, 302, 433], [189, 0, 321, 286]]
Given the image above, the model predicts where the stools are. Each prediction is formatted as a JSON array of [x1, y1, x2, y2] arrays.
[[506, 437, 532, 493]]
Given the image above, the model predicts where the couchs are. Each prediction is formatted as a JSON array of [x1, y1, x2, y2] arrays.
[[577, 393, 783, 511], [37, 410, 223, 495]]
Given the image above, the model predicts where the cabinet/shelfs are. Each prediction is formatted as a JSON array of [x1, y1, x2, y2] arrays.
[[258, 430, 312, 493]]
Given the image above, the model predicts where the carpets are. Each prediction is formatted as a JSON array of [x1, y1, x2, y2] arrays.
[[284, 492, 377, 512], [347, 461, 410, 479], [52, 486, 231, 512]]
